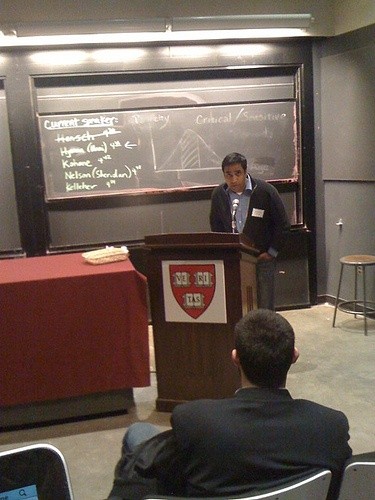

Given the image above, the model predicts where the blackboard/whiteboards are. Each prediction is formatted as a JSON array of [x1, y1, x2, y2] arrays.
[[37, 97, 299, 207]]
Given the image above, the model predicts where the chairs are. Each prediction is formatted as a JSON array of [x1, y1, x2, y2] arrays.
[[212, 451, 375, 500]]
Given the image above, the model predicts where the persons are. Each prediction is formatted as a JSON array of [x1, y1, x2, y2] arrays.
[[210, 152, 292, 311], [106, 309, 353, 500]]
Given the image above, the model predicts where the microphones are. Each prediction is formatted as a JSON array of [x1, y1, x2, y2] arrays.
[[232, 199, 239, 211]]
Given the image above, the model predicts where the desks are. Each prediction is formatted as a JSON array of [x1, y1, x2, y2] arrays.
[[0, 252, 149, 431]]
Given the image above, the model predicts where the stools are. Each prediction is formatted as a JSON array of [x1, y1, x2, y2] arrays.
[[332, 256, 375, 336]]
